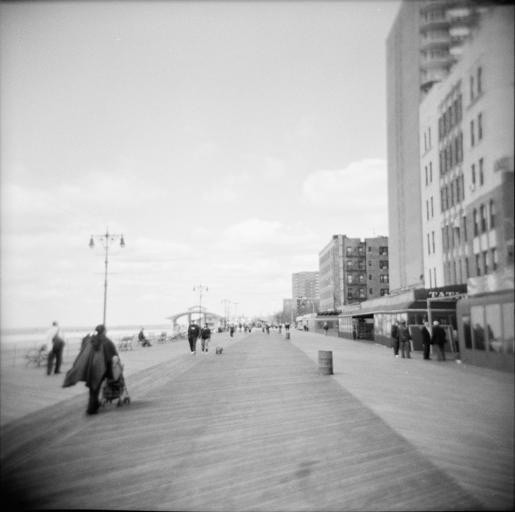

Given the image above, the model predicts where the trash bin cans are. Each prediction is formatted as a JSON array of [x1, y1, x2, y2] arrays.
[[317, 350, 333, 375], [285, 333, 290, 339]]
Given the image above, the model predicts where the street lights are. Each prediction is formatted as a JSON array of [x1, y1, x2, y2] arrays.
[[89, 227, 125, 326], [194, 284, 208, 338]]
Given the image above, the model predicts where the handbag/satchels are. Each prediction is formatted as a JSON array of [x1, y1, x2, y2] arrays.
[[53, 337, 65, 353]]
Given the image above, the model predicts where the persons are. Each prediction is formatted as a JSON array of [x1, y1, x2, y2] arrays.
[[138, 328, 152, 347], [218, 321, 309, 338], [37, 321, 64, 375], [86, 325, 118, 415], [323, 321, 328, 335], [430, 321, 447, 361], [391, 320, 400, 357], [188, 319, 199, 354], [199, 322, 211, 353], [421, 322, 432, 360], [396, 321, 413, 358]]
